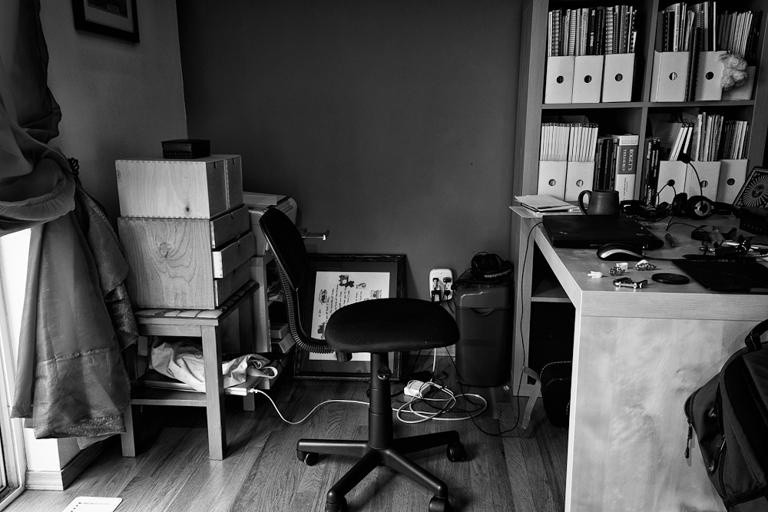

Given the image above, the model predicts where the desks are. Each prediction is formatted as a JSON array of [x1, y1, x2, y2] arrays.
[[513, 208, 768, 512], [112, 279, 258, 462]]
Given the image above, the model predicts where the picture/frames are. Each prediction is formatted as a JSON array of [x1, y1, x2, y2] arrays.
[[293, 252, 406, 382], [71, 1, 140, 44]]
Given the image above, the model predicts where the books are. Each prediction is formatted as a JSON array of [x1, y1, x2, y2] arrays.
[[657, 110, 751, 186], [508, 191, 589, 219], [541, 122, 617, 192], [547, 5, 637, 82], [656, 0, 752, 103], [641, 137, 660, 203]]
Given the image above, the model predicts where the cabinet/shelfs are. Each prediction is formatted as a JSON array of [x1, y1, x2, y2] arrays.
[[512, 1, 768, 397], [236, 250, 294, 390]]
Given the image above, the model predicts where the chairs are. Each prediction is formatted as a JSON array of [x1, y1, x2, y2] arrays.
[[258, 205, 467, 511]]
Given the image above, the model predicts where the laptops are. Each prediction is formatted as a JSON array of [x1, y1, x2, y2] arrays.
[[543, 215, 664, 251], [137, 367, 276, 397]]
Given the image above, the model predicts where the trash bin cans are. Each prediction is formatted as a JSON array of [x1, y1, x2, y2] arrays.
[[453, 263, 514, 388]]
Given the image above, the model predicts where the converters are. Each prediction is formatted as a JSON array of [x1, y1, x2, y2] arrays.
[[404, 380, 430, 398]]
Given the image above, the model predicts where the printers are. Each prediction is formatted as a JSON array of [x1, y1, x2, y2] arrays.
[[242, 193, 298, 256]]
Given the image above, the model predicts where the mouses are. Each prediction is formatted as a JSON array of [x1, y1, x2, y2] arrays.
[[597, 242, 642, 262]]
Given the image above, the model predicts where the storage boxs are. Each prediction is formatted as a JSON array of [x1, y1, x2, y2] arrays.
[[115, 154, 255, 312]]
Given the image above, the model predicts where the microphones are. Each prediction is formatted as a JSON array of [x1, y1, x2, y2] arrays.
[[650, 180, 674, 203], [682, 154, 702, 195]]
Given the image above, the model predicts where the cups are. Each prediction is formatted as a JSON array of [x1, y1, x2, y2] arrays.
[[578, 190, 622, 218]]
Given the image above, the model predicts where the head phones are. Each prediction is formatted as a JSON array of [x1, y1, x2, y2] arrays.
[[674, 194, 732, 220]]
